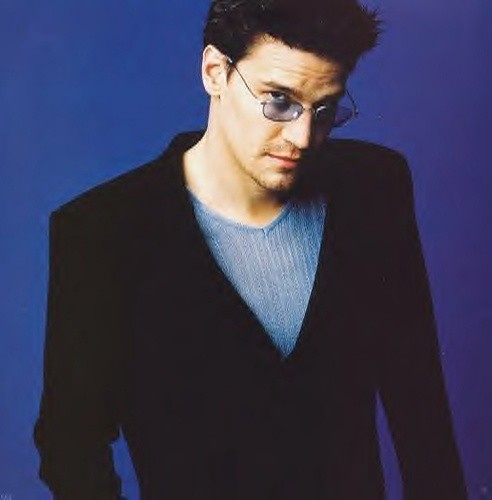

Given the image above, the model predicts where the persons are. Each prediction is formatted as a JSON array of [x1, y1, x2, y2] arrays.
[[34, 0, 468, 500]]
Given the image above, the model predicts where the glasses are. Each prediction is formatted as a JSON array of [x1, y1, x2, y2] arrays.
[[226, 56, 359, 129]]
